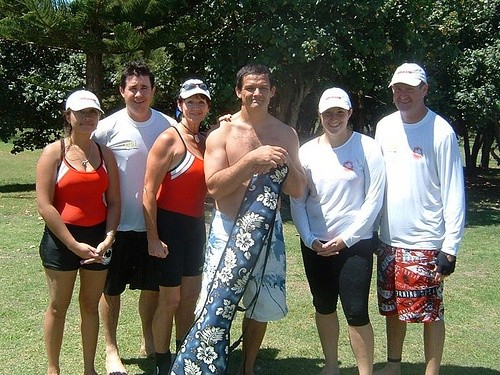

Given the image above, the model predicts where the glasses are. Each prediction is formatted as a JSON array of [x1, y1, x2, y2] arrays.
[[181, 83, 207, 91]]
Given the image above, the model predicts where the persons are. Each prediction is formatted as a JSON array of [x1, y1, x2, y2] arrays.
[[290, 87, 385, 375], [91, 65, 180, 375], [195, 64, 307, 375], [374, 63, 466, 375], [36, 90, 121, 375], [143, 79, 231, 375]]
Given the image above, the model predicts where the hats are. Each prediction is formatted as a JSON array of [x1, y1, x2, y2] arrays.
[[387, 62, 427, 88], [318, 87, 352, 114], [65, 90, 105, 115], [179, 78, 212, 101]]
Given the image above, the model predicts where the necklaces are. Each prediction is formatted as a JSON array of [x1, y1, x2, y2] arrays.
[[82, 161, 87, 165]]
